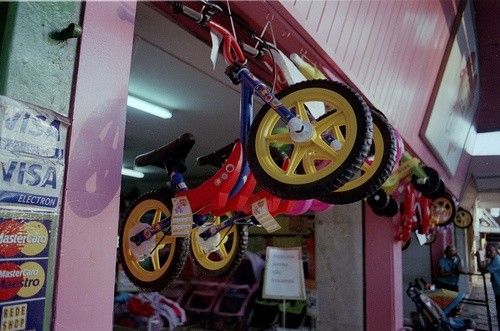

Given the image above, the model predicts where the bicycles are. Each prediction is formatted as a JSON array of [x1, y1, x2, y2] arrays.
[[190, 31, 397, 278], [286, 52, 474, 250], [116, 0, 373, 293]]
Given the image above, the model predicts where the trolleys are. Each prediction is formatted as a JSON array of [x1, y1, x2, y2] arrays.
[[406, 278, 478, 331]]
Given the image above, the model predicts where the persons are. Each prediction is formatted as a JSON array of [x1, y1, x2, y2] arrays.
[[436, 246, 462, 293], [475, 242, 500, 331]]
